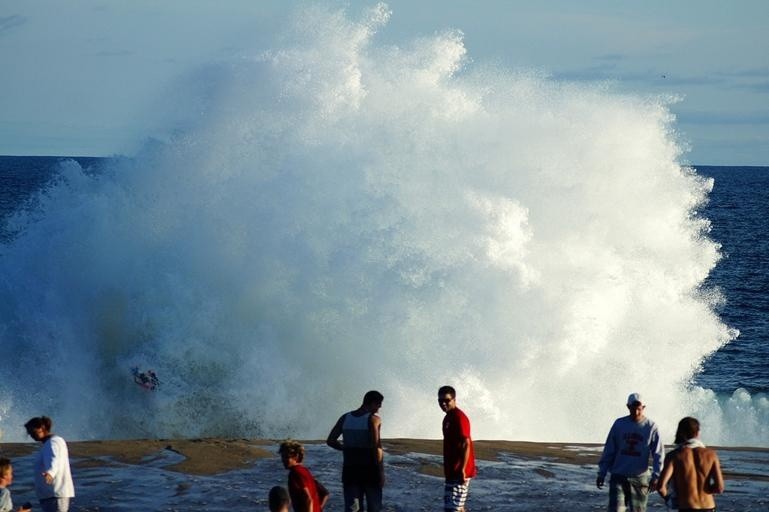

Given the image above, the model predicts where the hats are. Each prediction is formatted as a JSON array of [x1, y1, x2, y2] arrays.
[[628, 393, 644, 406]]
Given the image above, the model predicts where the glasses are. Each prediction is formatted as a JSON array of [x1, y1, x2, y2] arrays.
[[438, 399, 454, 403]]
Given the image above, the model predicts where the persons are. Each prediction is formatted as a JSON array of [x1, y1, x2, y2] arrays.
[[24, 416, 75, 512], [0, 459, 31, 512], [597, 394, 666, 512], [657, 418, 724, 512], [326, 390, 384, 512], [438, 386, 476, 512], [268, 440, 329, 512]]
